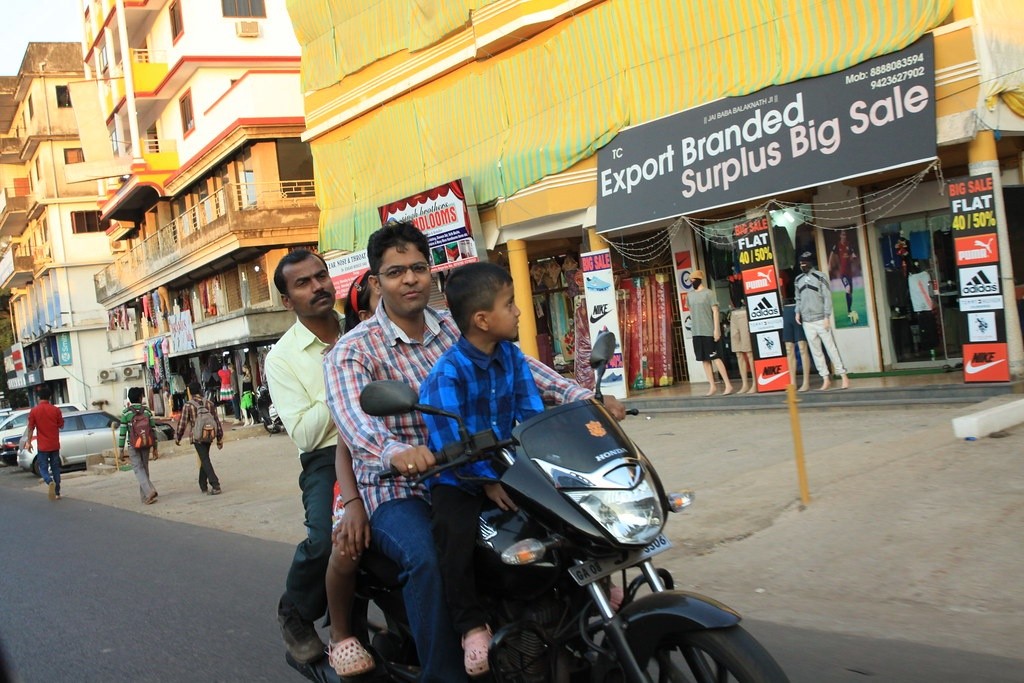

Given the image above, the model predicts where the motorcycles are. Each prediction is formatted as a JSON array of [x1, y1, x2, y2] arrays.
[[286, 331, 793, 683]]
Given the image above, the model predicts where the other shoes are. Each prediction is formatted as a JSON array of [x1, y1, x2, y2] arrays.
[[55, 495, 60, 499], [210, 487, 221, 495], [146, 490, 158, 501], [48, 481, 56, 500], [202, 488, 207, 494], [144, 498, 156, 505], [278, 613, 325, 664]]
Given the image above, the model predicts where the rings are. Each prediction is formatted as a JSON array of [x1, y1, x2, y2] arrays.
[[407, 463, 416, 469]]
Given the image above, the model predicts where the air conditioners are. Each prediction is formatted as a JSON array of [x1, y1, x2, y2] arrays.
[[237, 20, 260, 37], [122, 366, 139, 378], [98, 368, 116, 381]]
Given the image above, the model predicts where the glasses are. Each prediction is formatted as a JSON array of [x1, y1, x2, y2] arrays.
[[375, 261, 430, 279]]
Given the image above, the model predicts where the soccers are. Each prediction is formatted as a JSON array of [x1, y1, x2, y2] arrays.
[[850, 311, 859, 324]]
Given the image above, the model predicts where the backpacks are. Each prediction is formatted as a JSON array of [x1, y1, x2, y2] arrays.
[[130, 405, 154, 448], [188, 397, 217, 442]]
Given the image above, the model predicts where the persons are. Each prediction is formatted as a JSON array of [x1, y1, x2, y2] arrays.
[[783, 305, 811, 391], [241, 365, 254, 427], [325, 269, 384, 675], [728, 267, 756, 393], [228, 364, 240, 424], [27, 388, 64, 501], [118, 386, 158, 505], [829, 229, 856, 318], [686, 272, 733, 395], [897, 259, 941, 355], [176, 381, 224, 495], [418, 263, 545, 675], [265, 250, 347, 664], [135, 285, 171, 327], [325, 222, 627, 683], [794, 252, 849, 389]]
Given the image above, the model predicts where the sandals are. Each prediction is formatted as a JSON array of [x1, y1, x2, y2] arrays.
[[462, 623, 492, 677], [328, 636, 375, 676]]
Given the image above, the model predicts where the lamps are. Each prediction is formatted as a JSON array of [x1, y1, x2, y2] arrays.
[[781, 209, 795, 222], [23, 338, 30, 340], [32, 331, 37, 338], [795, 203, 800, 212], [46, 323, 53, 330]]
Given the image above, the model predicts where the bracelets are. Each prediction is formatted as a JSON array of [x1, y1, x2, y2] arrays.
[[343, 497, 364, 508]]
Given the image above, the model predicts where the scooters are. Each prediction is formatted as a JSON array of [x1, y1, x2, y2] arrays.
[[251, 380, 290, 438]]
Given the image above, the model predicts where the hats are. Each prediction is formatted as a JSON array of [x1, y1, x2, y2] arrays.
[[799, 251, 814, 262], [689, 270, 705, 280]]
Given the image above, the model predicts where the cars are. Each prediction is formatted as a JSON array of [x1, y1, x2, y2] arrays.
[[0, 402, 176, 479]]
